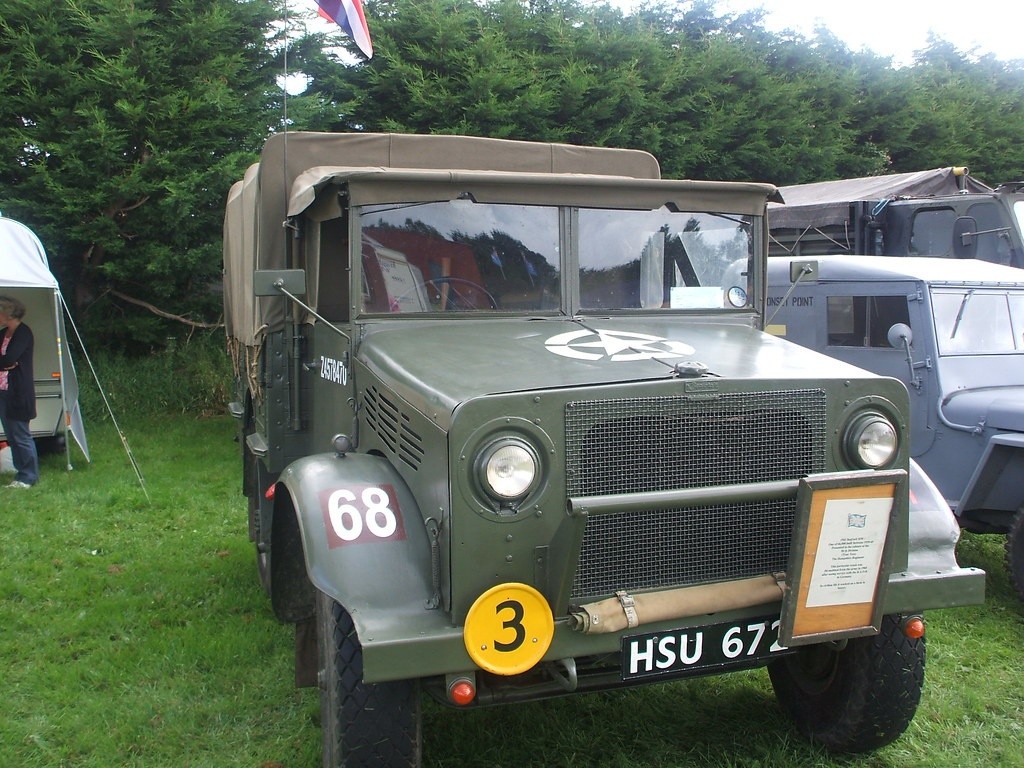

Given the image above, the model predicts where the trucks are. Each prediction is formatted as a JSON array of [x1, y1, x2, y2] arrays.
[[223, 130, 986, 768], [763, 165, 1023, 347]]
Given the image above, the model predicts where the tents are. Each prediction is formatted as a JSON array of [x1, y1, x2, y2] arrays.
[[1, 215, 91, 471]]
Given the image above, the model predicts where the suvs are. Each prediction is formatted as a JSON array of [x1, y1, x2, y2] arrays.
[[721, 255, 1024, 612]]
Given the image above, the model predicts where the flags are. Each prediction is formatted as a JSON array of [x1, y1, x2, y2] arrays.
[[316, 0, 372, 58]]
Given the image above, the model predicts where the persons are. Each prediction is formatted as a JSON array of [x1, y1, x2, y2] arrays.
[[1, 296, 40, 489]]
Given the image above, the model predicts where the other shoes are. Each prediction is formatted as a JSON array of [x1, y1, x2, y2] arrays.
[[4, 480, 32, 490]]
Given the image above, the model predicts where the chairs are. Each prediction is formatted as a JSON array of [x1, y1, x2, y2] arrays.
[[362, 226, 488, 312]]
